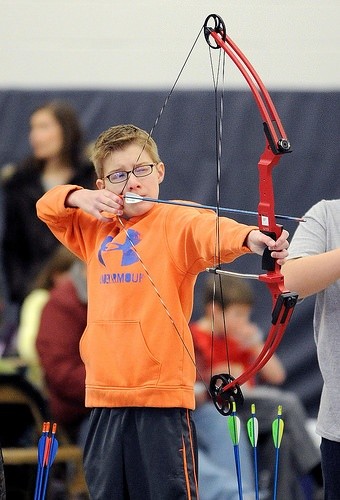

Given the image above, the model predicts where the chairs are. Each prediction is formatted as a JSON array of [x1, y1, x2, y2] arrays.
[[0, 374, 88, 500]]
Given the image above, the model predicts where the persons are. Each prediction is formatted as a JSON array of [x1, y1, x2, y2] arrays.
[[0, 105, 102, 331], [35, 122, 290, 500], [286, 199, 340, 500], [1, 242, 323, 500]]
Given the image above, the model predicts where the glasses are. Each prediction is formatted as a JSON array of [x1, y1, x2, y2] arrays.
[[100, 162, 157, 184]]
